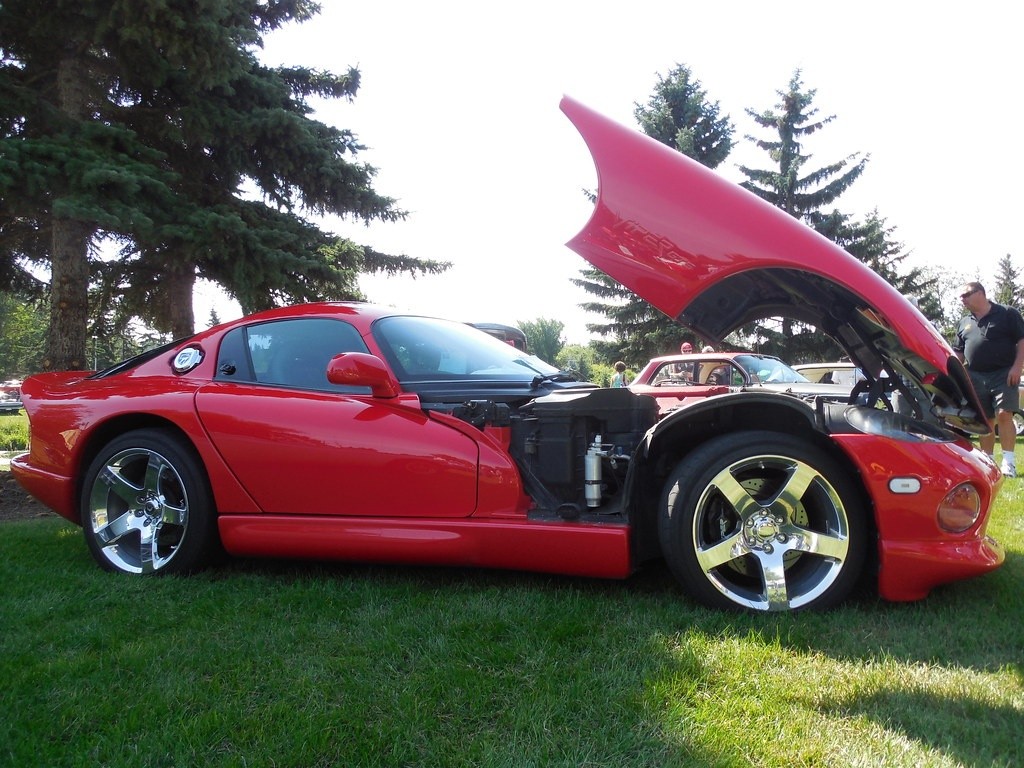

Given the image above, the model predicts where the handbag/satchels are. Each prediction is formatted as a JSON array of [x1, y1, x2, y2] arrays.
[[621, 374, 628, 387]]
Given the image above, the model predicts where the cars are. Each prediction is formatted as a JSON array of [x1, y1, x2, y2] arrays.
[[628, 353, 869, 419], [0, 381, 14, 387], [0, 391, 24, 413], [10, 94, 1005, 618], [762, 363, 890, 383]]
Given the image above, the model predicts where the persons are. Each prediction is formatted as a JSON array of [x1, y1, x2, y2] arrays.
[[954, 281, 1024, 476], [670, 342, 716, 384], [610, 361, 629, 388], [892, 294, 944, 427]]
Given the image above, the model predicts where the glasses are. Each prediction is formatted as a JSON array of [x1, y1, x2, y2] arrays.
[[960, 288, 979, 298], [682, 350, 692, 352]]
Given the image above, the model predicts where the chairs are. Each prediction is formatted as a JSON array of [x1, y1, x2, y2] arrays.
[[410, 341, 441, 372], [269, 340, 324, 391]]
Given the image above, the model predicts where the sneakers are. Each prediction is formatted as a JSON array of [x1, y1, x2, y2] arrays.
[[1001, 459, 1018, 477]]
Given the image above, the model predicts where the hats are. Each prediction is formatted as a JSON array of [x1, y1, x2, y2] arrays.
[[681, 342, 692, 348]]
